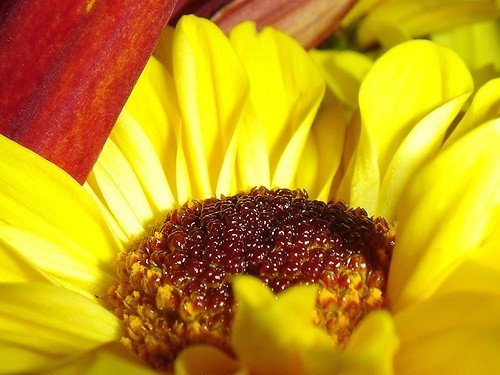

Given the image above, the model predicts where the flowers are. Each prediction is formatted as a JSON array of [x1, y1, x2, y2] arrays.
[[0, 0, 500, 375]]
